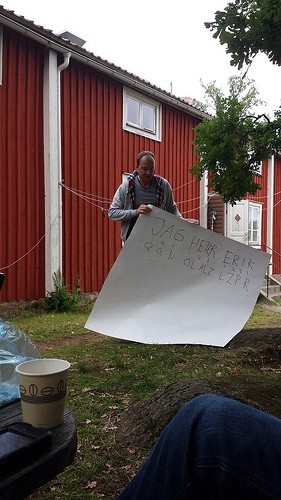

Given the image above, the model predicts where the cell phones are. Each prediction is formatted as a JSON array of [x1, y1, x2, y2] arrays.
[[0, 422, 53, 478]]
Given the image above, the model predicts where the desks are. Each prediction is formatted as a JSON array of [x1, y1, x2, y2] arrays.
[[0, 383, 79, 500]]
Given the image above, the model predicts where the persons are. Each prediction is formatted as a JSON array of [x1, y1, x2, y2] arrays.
[[107, 150, 200, 243], [116, 393, 281, 500]]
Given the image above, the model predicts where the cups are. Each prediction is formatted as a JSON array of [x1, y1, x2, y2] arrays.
[[16, 358, 71, 428]]
